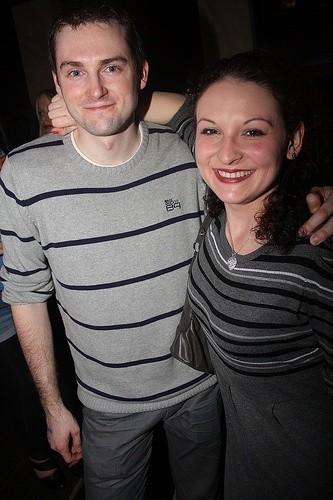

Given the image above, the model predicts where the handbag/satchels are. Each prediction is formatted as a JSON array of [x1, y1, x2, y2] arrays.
[[170, 213, 215, 374]]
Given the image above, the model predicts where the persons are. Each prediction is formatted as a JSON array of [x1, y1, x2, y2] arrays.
[[36, 89, 57, 137], [49, 68, 333, 500], [0, 0, 333, 500]]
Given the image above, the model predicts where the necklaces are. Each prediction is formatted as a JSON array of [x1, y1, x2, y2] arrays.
[[227, 217, 256, 271]]
